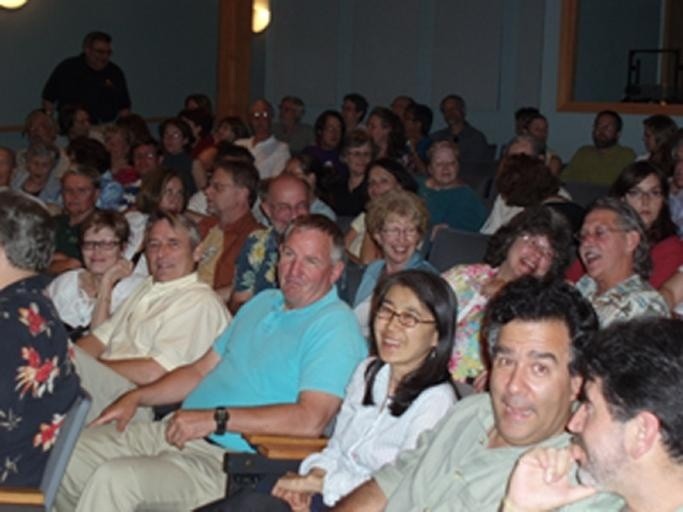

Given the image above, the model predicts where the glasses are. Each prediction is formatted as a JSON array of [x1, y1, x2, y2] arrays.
[[84, 241, 116, 250], [377, 304, 435, 326]]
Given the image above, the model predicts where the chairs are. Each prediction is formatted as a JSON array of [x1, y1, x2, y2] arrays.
[[1, 388, 92, 512], [224, 382, 480, 512], [485, 143, 498, 194], [340, 264, 364, 305], [427, 228, 491, 272]]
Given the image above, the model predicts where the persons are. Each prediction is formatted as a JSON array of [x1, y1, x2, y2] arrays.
[[39, 31, 133, 134], [3, 92, 681, 512]]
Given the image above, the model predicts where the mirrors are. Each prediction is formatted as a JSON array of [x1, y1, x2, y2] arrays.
[[556, 0, 682, 117]]
